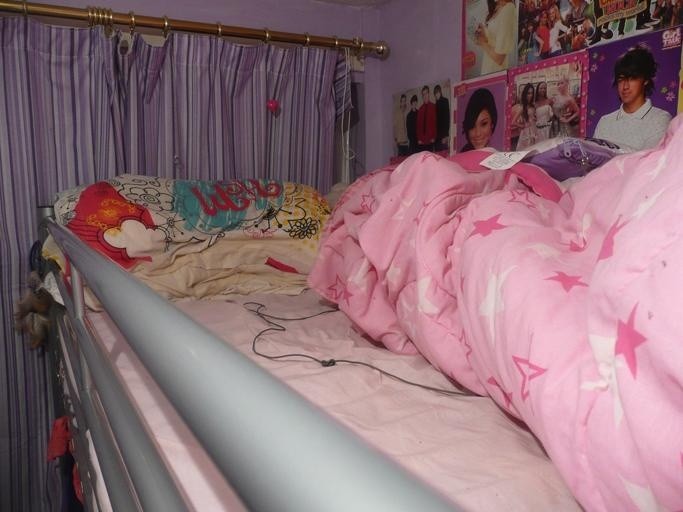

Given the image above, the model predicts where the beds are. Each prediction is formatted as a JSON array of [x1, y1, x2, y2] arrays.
[[13, 133, 682, 509]]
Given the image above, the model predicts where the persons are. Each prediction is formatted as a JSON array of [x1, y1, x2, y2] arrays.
[[552, 76, 580, 137], [513, 83, 537, 152], [392, 85, 450, 155], [458, 88, 498, 154], [593, 1, 681, 42], [477, 1, 515, 75], [533, 81, 555, 144], [517, 1, 596, 64], [592, 44, 672, 149]]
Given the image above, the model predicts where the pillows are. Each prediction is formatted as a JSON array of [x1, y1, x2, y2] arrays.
[[51, 176, 335, 310]]
